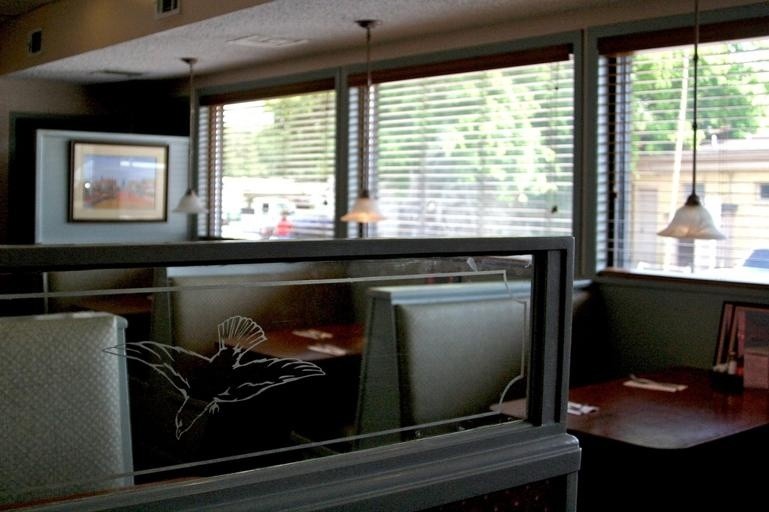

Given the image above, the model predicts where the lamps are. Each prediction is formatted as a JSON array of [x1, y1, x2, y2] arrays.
[[172, 58, 210, 214], [339, 18, 387, 223], [656, 0, 727, 239]]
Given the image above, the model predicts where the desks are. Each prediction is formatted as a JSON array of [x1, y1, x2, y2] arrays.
[[488, 362, 768, 511]]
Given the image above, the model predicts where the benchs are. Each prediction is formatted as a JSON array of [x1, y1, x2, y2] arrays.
[[1, 261, 594, 511]]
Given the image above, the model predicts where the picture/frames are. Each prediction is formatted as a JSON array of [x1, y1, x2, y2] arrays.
[[66, 140, 170, 224]]
